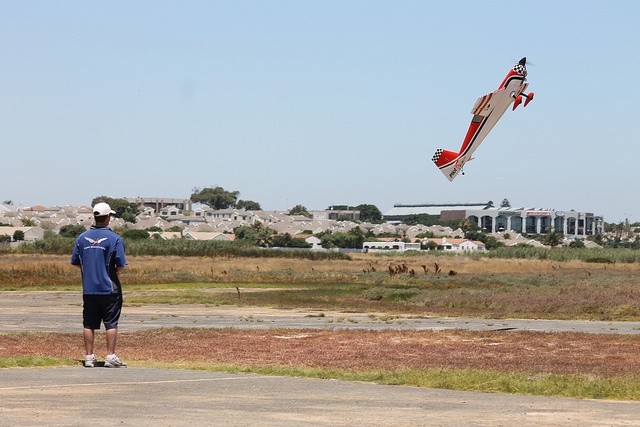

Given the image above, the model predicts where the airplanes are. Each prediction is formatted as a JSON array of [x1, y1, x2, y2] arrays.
[[430, 56, 534, 182]]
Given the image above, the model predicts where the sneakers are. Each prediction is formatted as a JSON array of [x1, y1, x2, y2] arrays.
[[84, 356, 99, 367], [104, 355, 127, 368]]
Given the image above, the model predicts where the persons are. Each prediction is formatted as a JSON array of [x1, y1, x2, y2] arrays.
[[69, 202, 128, 368]]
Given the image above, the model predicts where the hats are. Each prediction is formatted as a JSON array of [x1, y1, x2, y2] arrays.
[[93, 202, 116, 217]]
